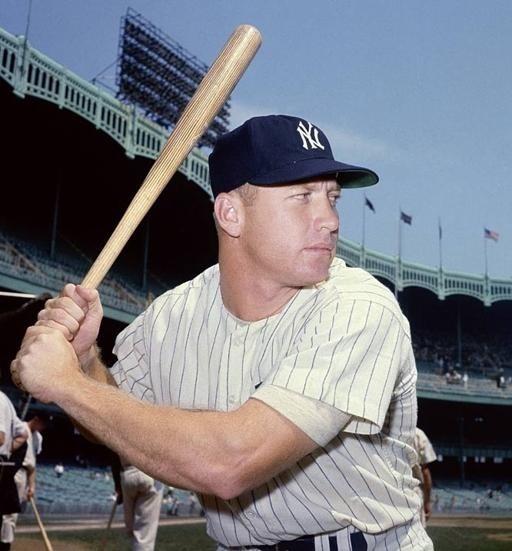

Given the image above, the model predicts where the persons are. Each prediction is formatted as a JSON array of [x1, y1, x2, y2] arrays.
[[0, 413, 48, 551], [414, 426, 438, 522], [109, 450, 164, 551], [0, 389, 28, 551], [8, 112, 436, 551]]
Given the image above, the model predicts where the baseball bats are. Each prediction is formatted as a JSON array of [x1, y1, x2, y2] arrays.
[[31, 495, 55, 551], [79, 25, 262, 287], [100, 500, 118, 550]]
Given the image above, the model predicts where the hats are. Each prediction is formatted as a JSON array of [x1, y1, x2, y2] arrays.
[[205, 112, 382, 198]]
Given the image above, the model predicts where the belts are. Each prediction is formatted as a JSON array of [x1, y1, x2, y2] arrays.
[[217, 528, 370, 551]]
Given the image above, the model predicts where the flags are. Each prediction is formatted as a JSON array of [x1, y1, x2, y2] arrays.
[[400, 212, 412, 225], [484, 228, 500, 242], [364, 195, 376, 212]]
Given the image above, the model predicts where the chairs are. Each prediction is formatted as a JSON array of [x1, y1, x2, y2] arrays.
[[22, 459, 200, 518], [1, 231, 177, 327], [417, 475, 510, 520], [404, 321, 511, 407]]
